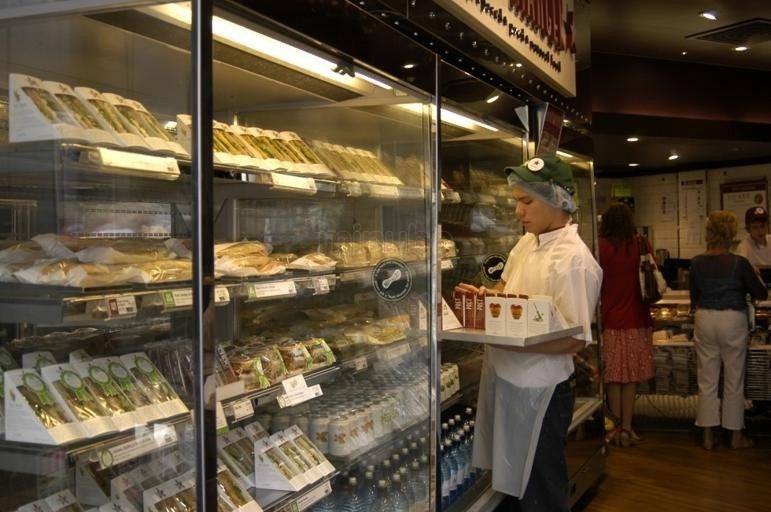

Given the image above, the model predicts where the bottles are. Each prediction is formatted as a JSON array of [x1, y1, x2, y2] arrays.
[[310, 402, 483, 512]]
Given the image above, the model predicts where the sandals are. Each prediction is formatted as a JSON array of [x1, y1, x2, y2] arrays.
[[730, 437, 756, 448], [611, 428, 620, 446], [704, 436, 721, 452], [620, 427, 648, 447]]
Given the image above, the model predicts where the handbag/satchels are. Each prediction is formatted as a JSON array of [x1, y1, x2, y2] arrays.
[[636, 233, 667, 304]]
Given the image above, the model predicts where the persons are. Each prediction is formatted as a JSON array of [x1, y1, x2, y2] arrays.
[[689, 210, 768, 451], [596, 201, 669, 446], [453, 154, 605, 511], [734, 206, 771, 267]]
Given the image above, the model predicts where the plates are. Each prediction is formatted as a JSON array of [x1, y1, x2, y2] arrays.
[[637, 345, 771, 401]]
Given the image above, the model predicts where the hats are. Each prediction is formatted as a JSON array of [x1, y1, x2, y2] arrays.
[[504, 152, 574, 195], [744, 205, 767, 223]]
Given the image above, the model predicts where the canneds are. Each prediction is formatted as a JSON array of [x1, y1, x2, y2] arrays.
[[253, 363, 460, 456]]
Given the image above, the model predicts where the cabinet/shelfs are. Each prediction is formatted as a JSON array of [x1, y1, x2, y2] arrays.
[[206, 1, 440, 509], [439, 50, 536, 510], [639, 286, 770, 422], [0, 1, 207, 512], [536, 112, 609, 512]]
